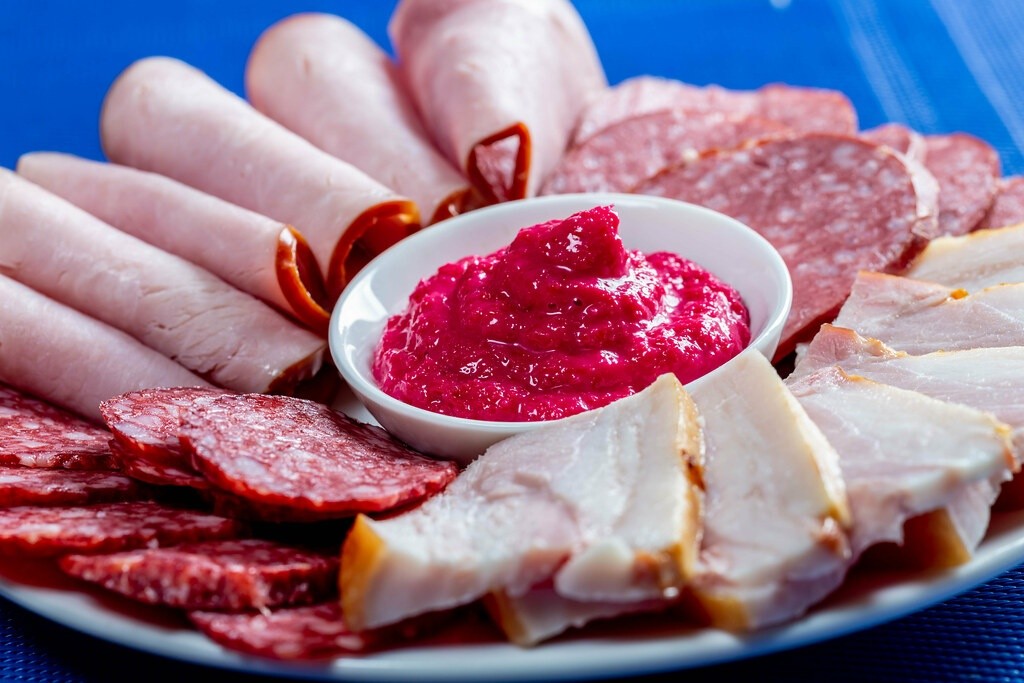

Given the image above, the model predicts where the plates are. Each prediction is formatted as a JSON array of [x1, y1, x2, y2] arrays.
[[1, 495, 1024, 682]]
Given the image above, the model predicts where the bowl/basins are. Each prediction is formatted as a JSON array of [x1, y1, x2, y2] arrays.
[[328, 192, 793, 473]]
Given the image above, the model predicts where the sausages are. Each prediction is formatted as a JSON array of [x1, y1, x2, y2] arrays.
[[541, 73, 1024, 361], [0, 388, 458, 658]]
